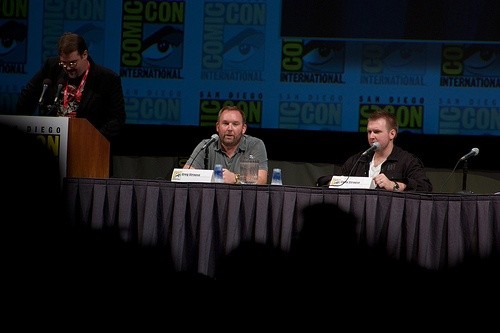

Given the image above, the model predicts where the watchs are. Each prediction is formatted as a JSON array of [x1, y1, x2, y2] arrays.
[[393, 181, 399, 192]]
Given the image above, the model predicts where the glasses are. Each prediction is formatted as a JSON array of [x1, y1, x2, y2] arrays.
[[59, 54, 83, 67]]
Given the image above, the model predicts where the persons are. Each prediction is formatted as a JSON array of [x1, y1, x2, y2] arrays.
[[326, 109, 433, 192], [182, 106, 267, 184], [15, 31, 127, 177]]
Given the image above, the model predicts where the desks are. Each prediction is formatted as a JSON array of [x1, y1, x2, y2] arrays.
[[65, 176, 500, 276]]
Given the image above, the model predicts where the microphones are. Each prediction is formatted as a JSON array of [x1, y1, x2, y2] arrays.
[[460, 147, 480, 161], [201, 134, 219, 150], [362, 142, 381, 156], [39, 79, 52, 104], [55, 78, 66, 103]]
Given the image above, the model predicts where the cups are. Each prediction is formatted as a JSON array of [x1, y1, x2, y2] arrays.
[[270, 168, 283, 185], [214, 163, 223, 182], [239, 159, 259, 184]]
